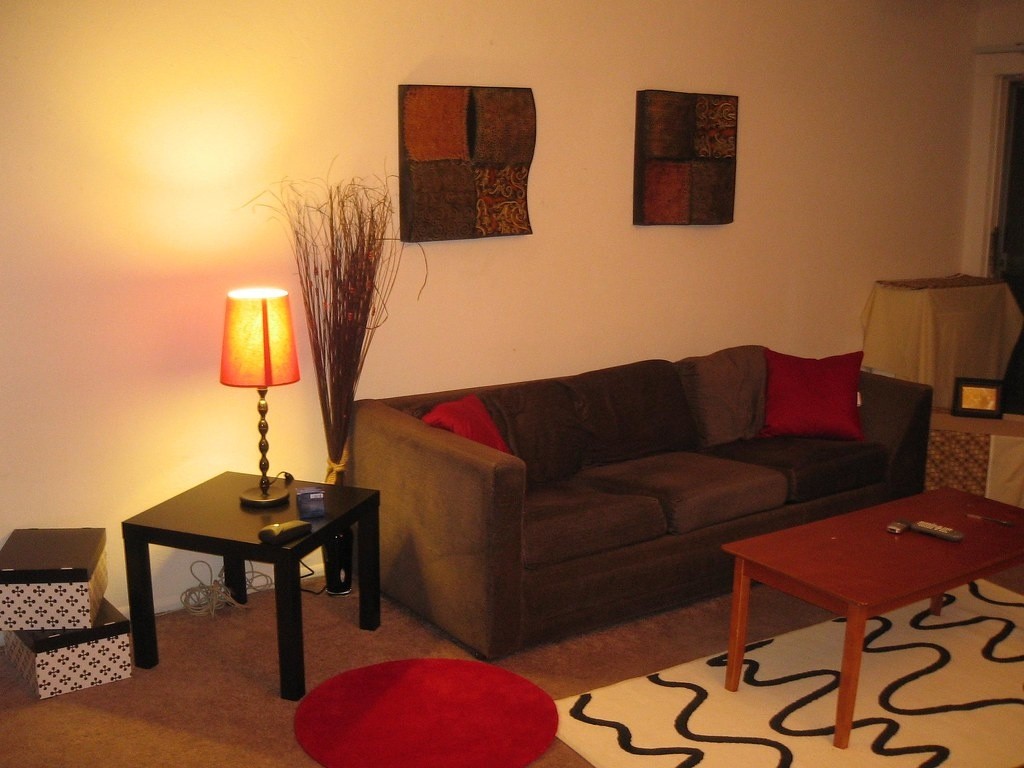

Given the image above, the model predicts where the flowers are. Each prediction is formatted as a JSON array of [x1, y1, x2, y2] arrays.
[[280, 174, 429, 462]]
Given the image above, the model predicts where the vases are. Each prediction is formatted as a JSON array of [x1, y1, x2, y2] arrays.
[[317, 530, 354, 595]]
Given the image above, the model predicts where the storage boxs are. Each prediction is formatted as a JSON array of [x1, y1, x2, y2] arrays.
[[0, 528, 108, 631], [3, 597, 131, 699]]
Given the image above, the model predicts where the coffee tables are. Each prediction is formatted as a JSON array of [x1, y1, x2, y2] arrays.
[[722, 485, 1024, 749]]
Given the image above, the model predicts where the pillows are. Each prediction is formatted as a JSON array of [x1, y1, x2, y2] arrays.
[[420, 395, 513, 455], [762, 347, 867, 440]]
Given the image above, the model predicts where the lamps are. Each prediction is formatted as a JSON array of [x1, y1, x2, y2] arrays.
[[219, 285, 301, 507]]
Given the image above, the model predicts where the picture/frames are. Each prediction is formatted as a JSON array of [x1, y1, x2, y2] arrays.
[[949, 376, 1005, 419]]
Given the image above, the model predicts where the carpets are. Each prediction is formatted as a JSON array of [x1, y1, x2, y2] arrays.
[[552, 578, 1024, 768]]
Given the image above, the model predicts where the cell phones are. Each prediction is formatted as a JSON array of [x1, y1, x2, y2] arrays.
[[888, 519, 911, 534]]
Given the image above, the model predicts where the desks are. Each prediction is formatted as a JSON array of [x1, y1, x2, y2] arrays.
[[292, 658, 559, 768], [122, 470, 382, 700], [859, 273, 1024, 407]]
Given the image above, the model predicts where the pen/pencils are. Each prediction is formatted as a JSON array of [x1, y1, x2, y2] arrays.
[[965, 513, 1009, 525]]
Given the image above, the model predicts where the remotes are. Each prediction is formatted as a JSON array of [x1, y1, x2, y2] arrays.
[[909, 521, 964, 541]]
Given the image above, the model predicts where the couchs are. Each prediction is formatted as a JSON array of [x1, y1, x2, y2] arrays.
[[343, 344, 932, 659]]
